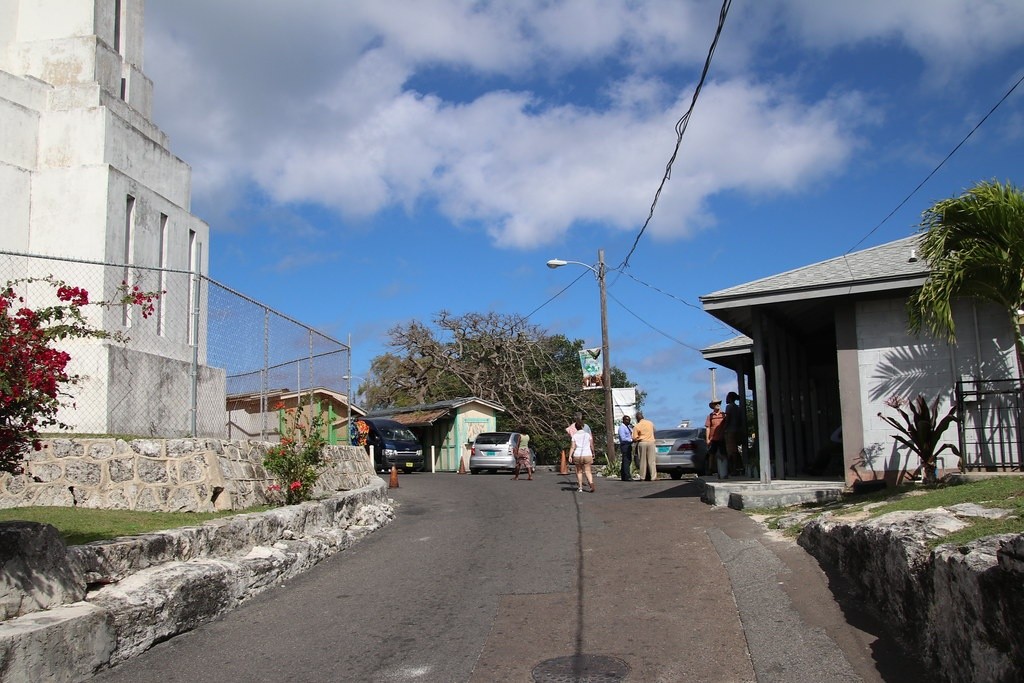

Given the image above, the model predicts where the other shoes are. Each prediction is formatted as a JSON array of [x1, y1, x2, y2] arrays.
[[623, 477, 634, 481], [577, 488, 583, 492], [589, 482, 595, 493], [512, 477, 518, 481]]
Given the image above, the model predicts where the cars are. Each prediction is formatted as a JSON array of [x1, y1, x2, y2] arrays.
[[634, 427, 717, 479], [469, 432, 536, 474]]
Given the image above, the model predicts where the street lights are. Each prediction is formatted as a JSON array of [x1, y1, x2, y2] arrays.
[[343, 375, 370, 411], [546, 249, 616, 463]]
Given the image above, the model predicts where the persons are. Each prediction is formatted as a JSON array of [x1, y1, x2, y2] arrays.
[[618, 415, 636, 482], [632, 412, 659, 481], [565, 411, 595, 484], [718, 392, 745, 480], [568, 420, 596, 494], [705, 398, 732, 480], [511, 426, 535, 480]]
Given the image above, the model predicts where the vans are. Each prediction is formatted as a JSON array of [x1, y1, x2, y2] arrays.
[[362, 419, 424, 473]]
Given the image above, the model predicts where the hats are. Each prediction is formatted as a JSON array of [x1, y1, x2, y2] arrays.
[[709, 398, 722, 408]]
